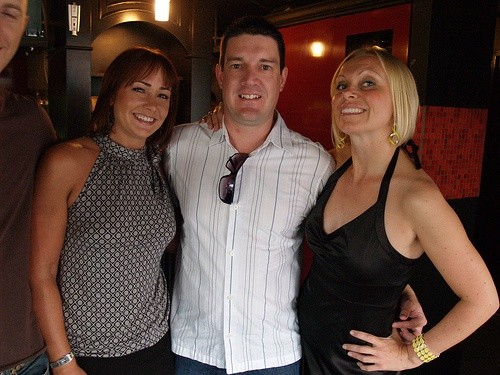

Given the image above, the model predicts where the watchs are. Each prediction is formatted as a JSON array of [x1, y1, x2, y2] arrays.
[[49, 348, 75, 369]]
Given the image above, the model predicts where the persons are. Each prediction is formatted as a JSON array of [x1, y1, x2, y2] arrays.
[[0, 0, 60, 375], [29, 47, 180, 375], [200, 46, 499, 375], [160, 14, 428, 375]]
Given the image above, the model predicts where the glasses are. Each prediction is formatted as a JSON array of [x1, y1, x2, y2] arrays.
[[219, 152, 250, 206]]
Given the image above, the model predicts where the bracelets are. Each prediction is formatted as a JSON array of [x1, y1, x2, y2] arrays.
[[412, 333, 440, 363]]
